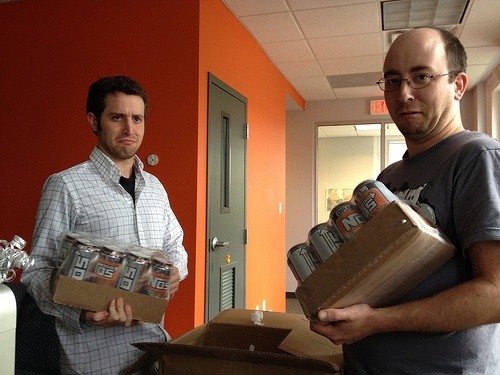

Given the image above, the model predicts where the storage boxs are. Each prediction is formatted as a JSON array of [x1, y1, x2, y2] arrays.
[[130, 309, 344, 375], [294, 200, 458, 325], [49, 268, 169, 324]]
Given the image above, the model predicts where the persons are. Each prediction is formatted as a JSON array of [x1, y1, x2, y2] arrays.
[[21, 75, 188, 375], [309, 26, 500, 375]]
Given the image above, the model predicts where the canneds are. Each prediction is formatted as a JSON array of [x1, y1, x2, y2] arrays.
[[58, 232, 173, 299], [286, 179, 399, 285]]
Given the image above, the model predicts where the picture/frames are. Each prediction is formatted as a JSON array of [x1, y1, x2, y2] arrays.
[[325, 187, 356, 213]]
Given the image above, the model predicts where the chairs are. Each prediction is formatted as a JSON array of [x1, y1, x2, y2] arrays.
[[2, 281, 61, 375]]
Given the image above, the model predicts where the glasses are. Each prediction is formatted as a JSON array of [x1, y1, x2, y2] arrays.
[[376, 72, 458, 91]]
